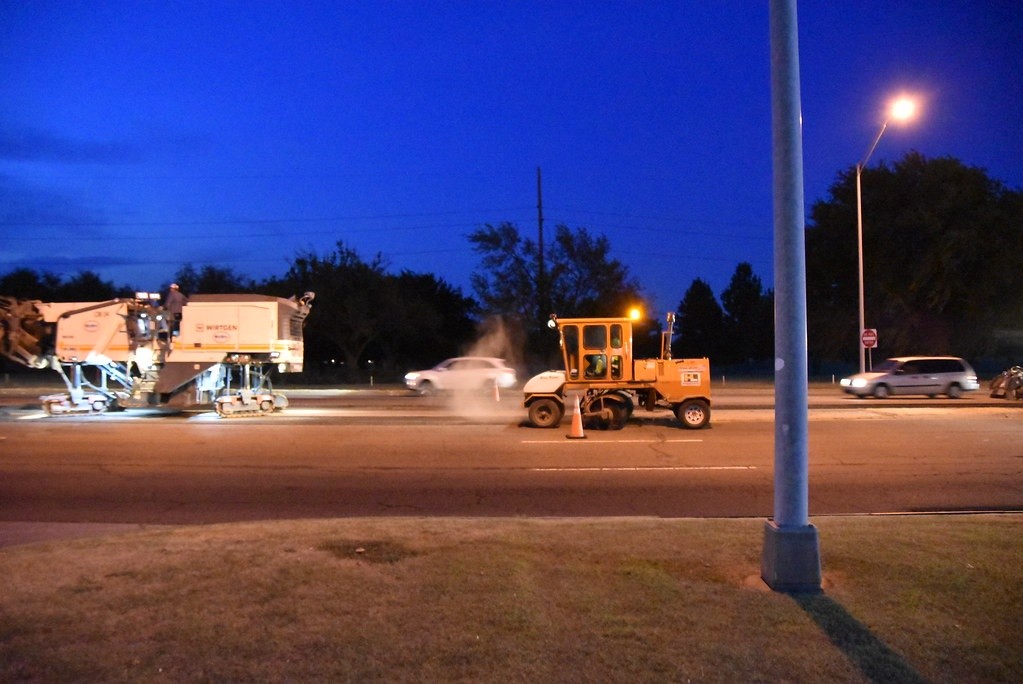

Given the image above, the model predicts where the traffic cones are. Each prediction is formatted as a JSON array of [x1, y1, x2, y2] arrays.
[[564, 395, 587, 441], [491, 378, 501, 405]]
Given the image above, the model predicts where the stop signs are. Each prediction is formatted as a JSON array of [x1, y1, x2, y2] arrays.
[[861, 330, 879, 348]]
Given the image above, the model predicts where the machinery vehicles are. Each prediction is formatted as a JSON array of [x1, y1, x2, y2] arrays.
[[523, 308, 713, 430], [1, 292, 313, 418]]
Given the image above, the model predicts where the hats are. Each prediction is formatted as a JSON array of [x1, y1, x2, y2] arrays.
[[170, 283, 179, 289]]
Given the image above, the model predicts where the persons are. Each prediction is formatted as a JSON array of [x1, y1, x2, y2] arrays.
[[166, 284, 186, 319], [151, 302, 171, 365], [586, 332, 620, 376]]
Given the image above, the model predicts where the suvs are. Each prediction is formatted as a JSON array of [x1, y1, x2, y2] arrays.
[[403, 355, 516, 395]]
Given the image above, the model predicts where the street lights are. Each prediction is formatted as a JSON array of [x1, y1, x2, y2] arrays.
[[857, 90, 915, 373]]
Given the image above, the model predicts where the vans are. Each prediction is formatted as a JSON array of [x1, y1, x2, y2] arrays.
[[838, 356, 979, 399]]
